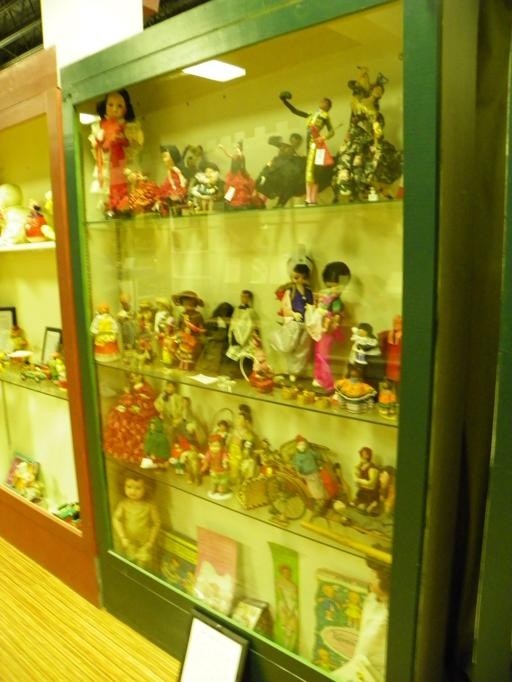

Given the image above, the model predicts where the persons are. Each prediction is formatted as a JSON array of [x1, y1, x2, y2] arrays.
[[90, 256, 401, 415], [112, 466, 161, 572], [88, 65, 388, 217], [102, 370, 395, 525], [0, 323, 68, 395], [86, 66, 404, 682]]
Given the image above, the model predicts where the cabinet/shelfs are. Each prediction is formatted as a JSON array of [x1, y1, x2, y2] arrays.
[[0, 2, 512, 682]]
